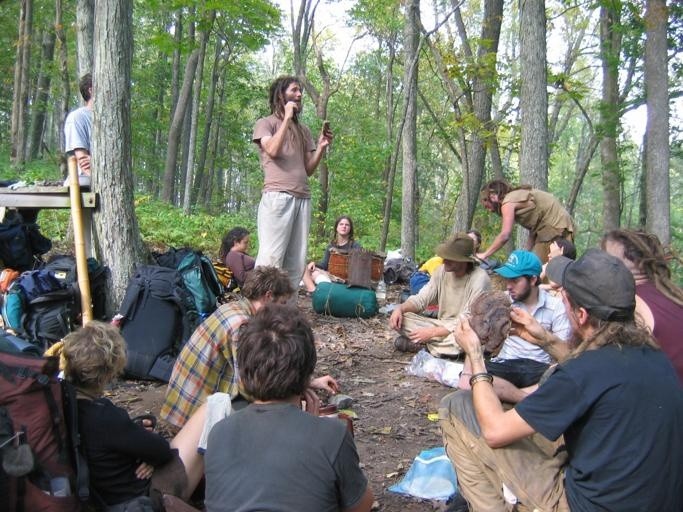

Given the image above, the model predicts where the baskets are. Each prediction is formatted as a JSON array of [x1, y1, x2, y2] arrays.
[[328, 251, 384, 290]]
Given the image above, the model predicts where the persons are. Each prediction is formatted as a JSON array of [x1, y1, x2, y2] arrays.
[[218, 227, 257, 287], [469, 178, 574, 264], [0, 207, 51, 272], [481, 251, 574, 389], [388, 235, 491, 357], [303, 216, 361, 291], [437, 247, 682, 510], [58, 266, 375, 512], [64, 73, 97, 262], [253, 75, 333, 306], [600, 227, 683, 378]]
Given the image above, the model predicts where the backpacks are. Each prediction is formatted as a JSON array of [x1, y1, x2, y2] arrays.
[[117, 248, 225, 382], [1, 350, 83, 512], [3, 252, 106, 351]]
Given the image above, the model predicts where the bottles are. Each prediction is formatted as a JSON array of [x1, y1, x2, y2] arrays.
[[375, 281, 385, 304]]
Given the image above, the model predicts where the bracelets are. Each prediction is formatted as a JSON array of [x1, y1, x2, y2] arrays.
[[469, 373, 495, 391]]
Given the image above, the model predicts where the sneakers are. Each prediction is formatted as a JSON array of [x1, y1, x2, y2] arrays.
[[394, 335, 430, 353]]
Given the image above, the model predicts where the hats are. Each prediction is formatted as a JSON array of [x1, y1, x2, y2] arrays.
[[434, 233, 481, 266], [545, 248, 635, 321], [493, 250, 542, 279]]
